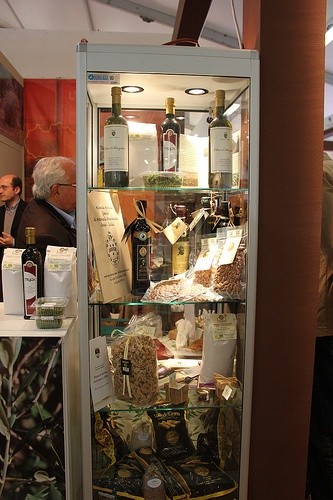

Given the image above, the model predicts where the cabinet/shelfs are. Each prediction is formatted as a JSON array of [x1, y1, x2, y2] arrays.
[[0, 302, 82, 500], [75, 37, 262, 498]]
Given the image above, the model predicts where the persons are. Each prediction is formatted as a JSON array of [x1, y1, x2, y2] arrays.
[[17, 157, 76, 261], [304, 152, 333, 500], [0, 174, 28, 302]]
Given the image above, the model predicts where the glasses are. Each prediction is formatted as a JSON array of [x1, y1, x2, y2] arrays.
[[57, 183, 76, 186], [1, 185, 18, 189]]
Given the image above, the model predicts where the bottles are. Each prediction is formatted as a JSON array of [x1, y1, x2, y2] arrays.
[[104, 87, 129, 188], [22, 226, 43, 320], [172, 197, 242, 276], [208, 89, 233, 187], [131, 199, 151, 296], [161, 98, 180, 173]]
